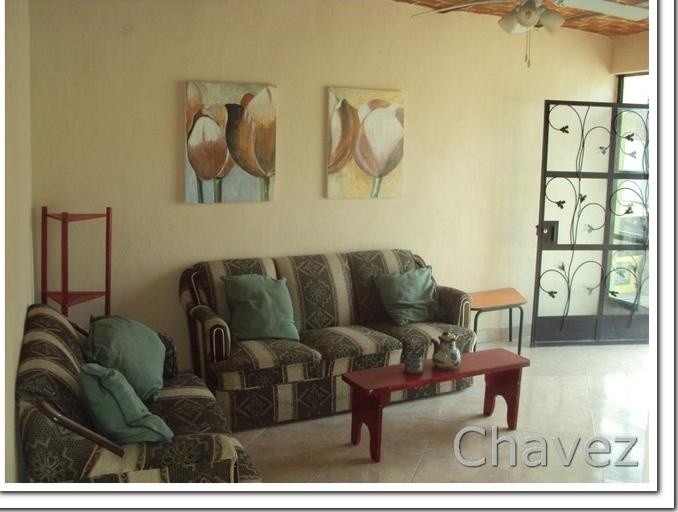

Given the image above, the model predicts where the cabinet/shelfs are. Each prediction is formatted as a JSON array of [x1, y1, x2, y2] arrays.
[[41, 206, 111, 317]]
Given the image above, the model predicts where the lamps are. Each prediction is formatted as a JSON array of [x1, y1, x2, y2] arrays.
[[498, 0, 566, 34]]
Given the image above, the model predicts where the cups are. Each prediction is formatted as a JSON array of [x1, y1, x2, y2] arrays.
[[404, 352, 424, 376]]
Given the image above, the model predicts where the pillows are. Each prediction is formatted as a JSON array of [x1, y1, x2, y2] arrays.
[[371, 265, 450, 328], [77, 314, 175, 442], [219, 273, 301, 341]]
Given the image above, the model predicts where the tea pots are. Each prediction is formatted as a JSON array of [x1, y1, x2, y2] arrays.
[[430, 331, 462, 370]]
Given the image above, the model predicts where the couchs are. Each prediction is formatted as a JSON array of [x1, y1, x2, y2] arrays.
[[179, 248, 479, 431], [15, 304, 263, 483]]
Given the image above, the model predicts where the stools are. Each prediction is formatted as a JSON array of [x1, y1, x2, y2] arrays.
[[468, 287, 527, 356]]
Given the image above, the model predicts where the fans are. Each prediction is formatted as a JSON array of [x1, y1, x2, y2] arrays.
[[412, 0, 647, 66]]
